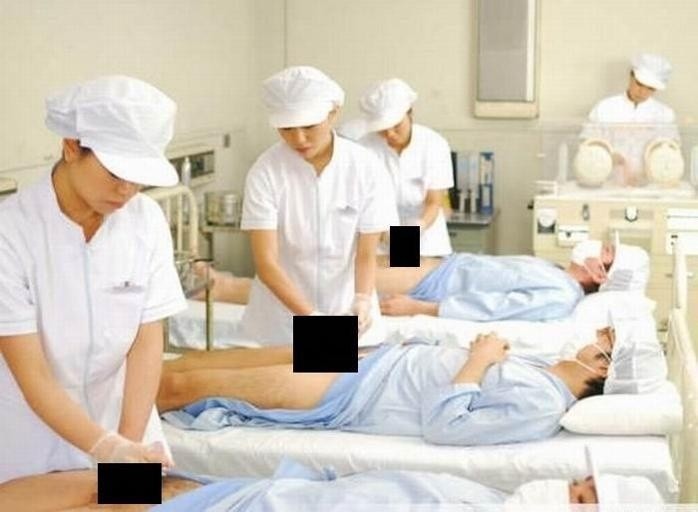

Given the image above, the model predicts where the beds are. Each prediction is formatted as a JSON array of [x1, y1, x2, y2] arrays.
[[155, 235, 698, 512]]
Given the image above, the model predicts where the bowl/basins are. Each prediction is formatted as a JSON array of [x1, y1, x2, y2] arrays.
[[206, 189, 241, 228]]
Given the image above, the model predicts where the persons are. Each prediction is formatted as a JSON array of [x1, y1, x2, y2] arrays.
[[154, 307, 668, 447], [0, 76, 188, 483], [577, 54, 683, 189], [352, 76, 453, 259], [188, 228, 651, 323], [0, 470, 663, 512], [225, 65, 400, 348]]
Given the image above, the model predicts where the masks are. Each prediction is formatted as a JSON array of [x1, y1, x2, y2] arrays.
[[504, 478, 572, 511], [570, 239, 607, 283], [558, 324, 609, 377]]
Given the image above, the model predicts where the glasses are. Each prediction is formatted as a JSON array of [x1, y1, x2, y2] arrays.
[[598, 326, 614, 350]]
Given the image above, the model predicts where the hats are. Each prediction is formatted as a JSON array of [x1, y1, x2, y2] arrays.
[[583, 446, 661, 506], [359, 78, 419, 134], [41, 74, 179, 188], [602, 302, 668, 395], [258, 65, 345, 130], [598, 227, 650, 292], [627, 52, 672, 92]]
